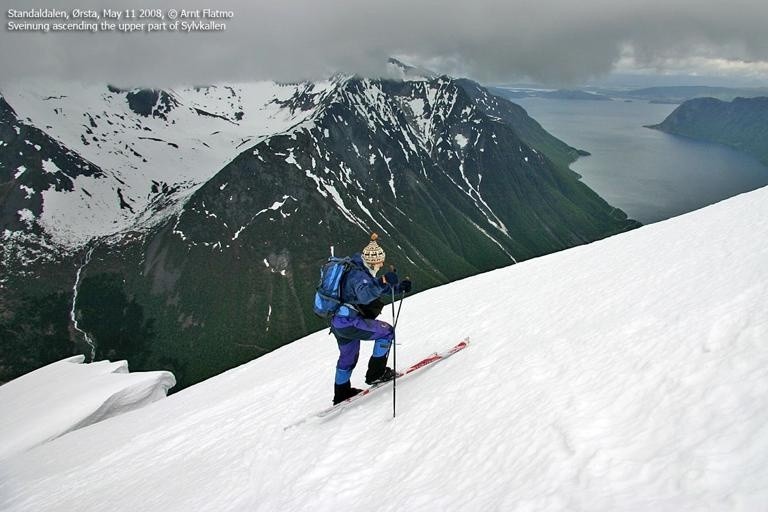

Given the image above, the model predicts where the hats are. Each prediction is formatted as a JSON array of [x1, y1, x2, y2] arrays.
[[360, 240, 385, 264]]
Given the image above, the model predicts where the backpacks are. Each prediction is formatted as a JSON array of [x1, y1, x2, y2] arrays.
[[312, 256, 358, 320]]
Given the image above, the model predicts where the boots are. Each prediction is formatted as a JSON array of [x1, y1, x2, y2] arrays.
[[332, 380, 364, 406], [365, 348, 400, 385]]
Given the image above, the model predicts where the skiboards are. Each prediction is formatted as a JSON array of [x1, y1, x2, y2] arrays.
[[284, 336, 468, 431]]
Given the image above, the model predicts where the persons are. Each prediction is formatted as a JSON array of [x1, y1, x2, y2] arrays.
[[331, 233, 411, 406]]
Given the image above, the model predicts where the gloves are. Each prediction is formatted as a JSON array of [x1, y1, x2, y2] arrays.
[[381, 271, 412, 293]]
